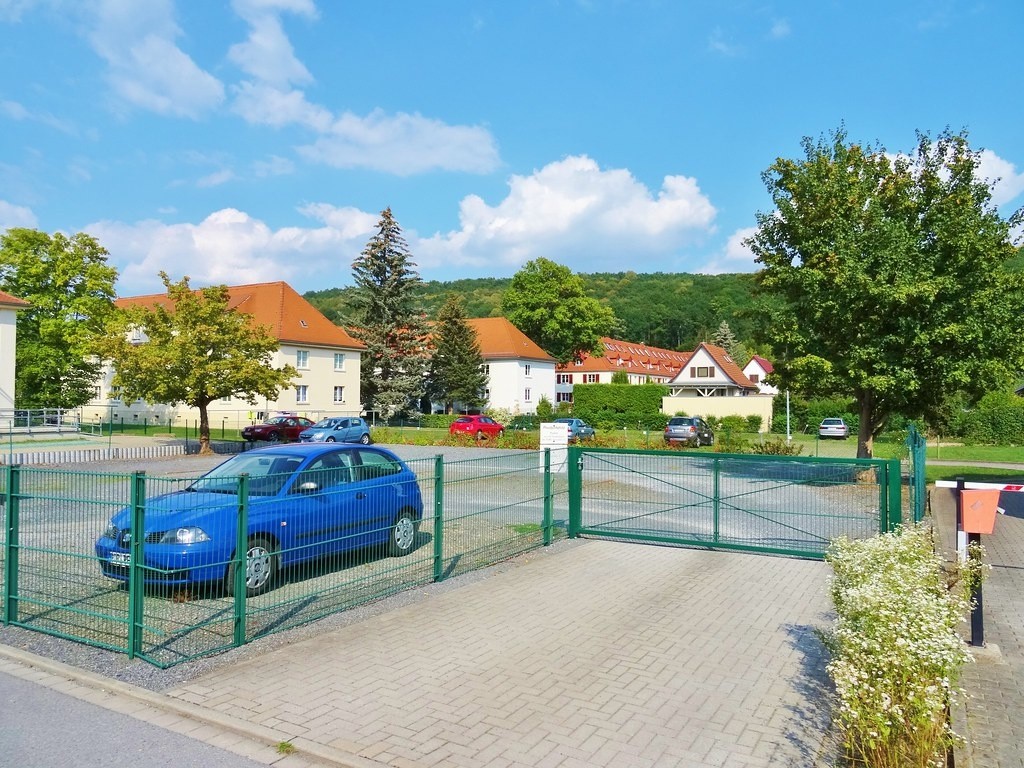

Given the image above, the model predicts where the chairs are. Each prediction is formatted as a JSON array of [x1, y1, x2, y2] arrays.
[[278, 461, 299, 485]]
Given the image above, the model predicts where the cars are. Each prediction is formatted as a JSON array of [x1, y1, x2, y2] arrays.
[[298, 416, 370, 445], [663, 416, 714, 448], [819, 417, 849, 440], [552, 418, 595, 443], [448, 415, 505, 441], [94, 442, 425, 598], [240, 416, 317, 441]]
